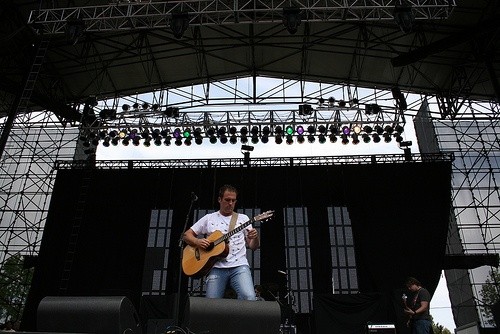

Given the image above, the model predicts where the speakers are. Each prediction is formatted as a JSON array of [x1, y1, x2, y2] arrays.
[[184, 296, 281, 334], [37, 296, 141, 334]]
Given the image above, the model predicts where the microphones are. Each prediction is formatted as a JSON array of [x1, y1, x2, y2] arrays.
[[192, 192, 198, 201]]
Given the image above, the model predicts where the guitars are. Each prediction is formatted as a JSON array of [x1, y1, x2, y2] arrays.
[[401, 293, 416, 329], [181, 210, 275, 279]]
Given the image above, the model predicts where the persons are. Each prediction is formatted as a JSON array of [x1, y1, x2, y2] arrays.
[[403, 276, 432, 334], [184, 184, 258, 301]]
